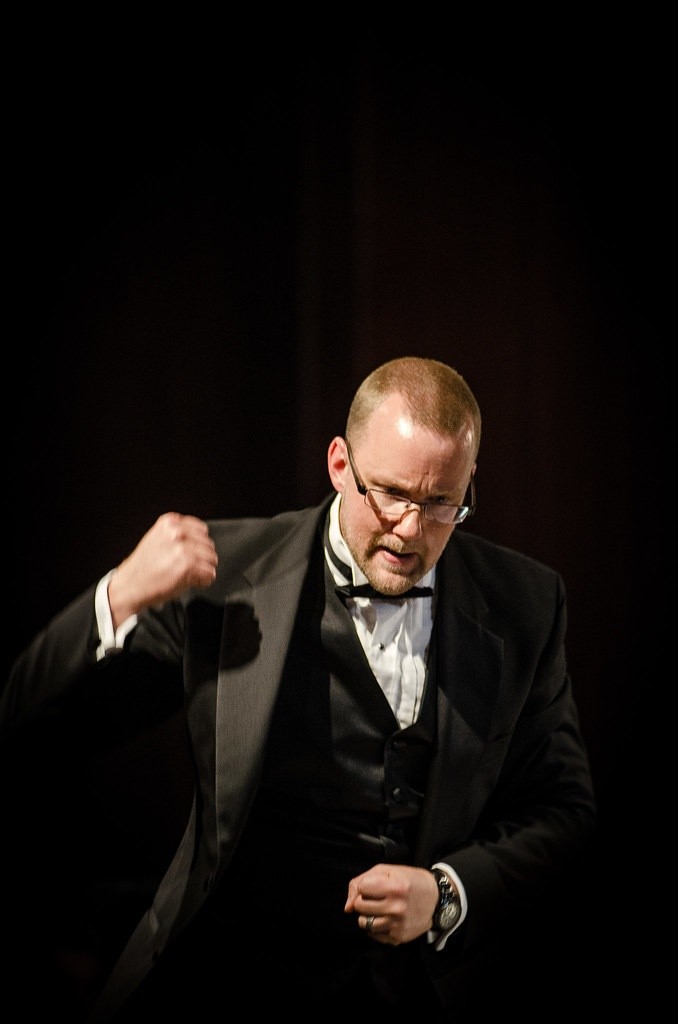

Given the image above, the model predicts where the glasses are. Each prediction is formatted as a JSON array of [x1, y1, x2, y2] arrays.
[[345, 438, 477, 525]]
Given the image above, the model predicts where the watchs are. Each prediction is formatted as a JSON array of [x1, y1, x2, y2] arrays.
[[429, 868, 461, 945]]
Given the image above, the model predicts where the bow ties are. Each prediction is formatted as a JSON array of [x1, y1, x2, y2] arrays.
[[322, 511, 435, 599]]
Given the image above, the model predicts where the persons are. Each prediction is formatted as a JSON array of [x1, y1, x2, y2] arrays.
[[0, 358, 596, 1024]]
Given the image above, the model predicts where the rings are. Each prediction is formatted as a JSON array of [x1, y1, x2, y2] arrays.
[[365, 916, 375, 932]]
